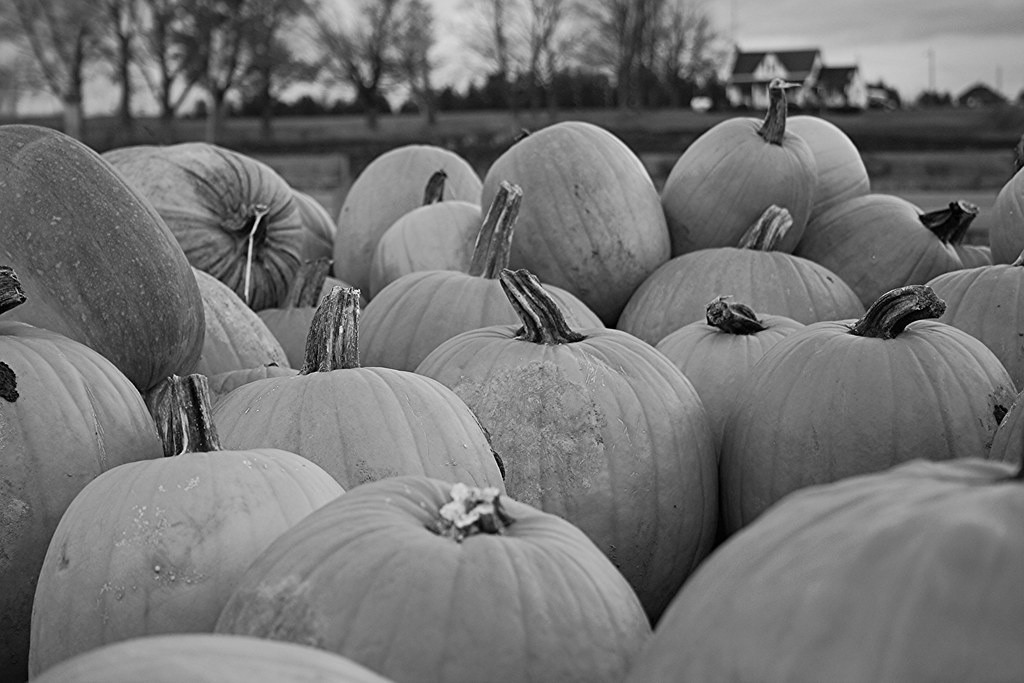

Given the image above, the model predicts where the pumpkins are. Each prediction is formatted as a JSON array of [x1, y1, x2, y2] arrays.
[[0, 77, 1024, 683]]
